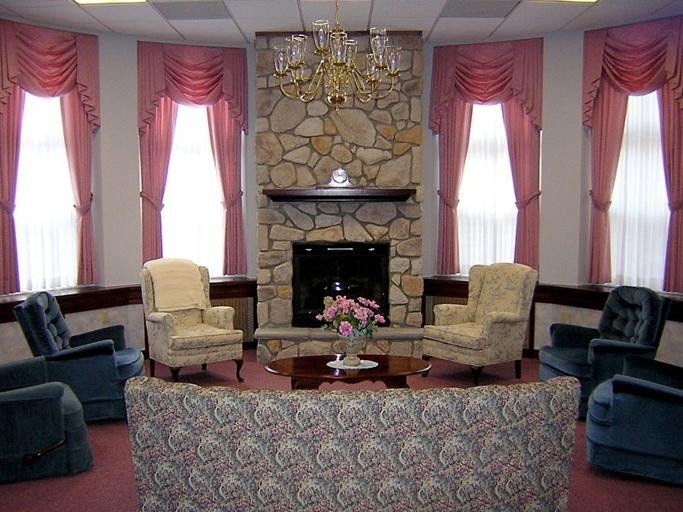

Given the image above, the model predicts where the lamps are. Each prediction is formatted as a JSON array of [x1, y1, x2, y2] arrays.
[[264, 0, 414, 101]]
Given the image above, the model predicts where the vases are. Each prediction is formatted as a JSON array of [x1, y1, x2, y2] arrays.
[[336, 328, 366, 365]]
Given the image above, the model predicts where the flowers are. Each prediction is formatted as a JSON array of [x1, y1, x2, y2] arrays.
[[316, 292, 387, 339]]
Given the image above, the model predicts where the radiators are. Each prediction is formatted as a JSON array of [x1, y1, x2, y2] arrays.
[[422, 295, 530, 351], [209, 296, 255, 342]]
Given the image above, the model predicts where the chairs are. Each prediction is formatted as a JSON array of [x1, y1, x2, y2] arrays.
[[122, 371, 585, 512], [537, 286, 670, 413], [139, 257, 249, 383], [583, 357, 680, 484], [417, 259, 543, 381], [13, 291, 146, 422], [0, 355, 93, 481]]
